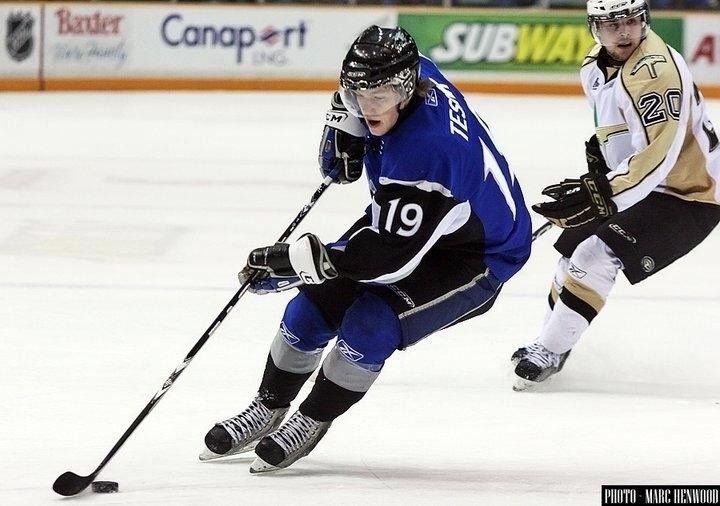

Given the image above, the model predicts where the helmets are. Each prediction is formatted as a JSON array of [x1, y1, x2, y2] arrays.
[[587, 0, 651, 25], [340, 25, 421, 99]]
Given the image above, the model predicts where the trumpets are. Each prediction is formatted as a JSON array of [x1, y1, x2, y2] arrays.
[[53, 161, 345, 496]]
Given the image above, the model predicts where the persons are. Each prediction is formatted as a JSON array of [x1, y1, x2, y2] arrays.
[[204, 25, 532, 470], [511, 0, 720, 383]]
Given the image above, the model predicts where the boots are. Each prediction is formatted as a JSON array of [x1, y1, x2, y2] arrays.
[[510, 339, 572, 382], [204, 394, 291, 455], [255, 408, 332, 468]]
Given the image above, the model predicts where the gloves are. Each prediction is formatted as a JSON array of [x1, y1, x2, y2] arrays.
[[237, 232, 338, 295], [531, 171, 618, 229], [318, 90, 368, 185]]
